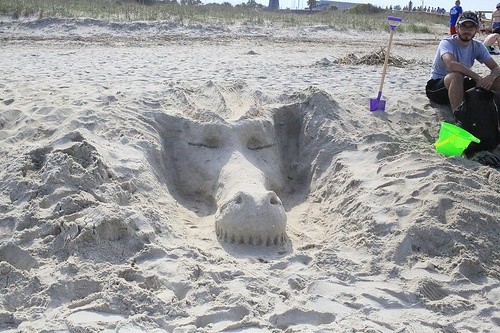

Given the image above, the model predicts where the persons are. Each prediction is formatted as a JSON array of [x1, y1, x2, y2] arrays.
[[491, 3, 500, 29], [426, 11, 500, 129], [450, 0, 463, 35], [385, 5, 446, 12]]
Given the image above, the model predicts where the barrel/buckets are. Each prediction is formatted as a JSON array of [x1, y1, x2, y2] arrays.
[[435, 121, 480, 156]]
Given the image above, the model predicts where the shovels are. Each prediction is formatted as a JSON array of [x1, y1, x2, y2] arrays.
[[370, 17, 402, 112]]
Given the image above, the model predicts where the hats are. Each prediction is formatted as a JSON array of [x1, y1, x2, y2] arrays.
[[496, 3, 500, 8], [454, 11, 480, 26]]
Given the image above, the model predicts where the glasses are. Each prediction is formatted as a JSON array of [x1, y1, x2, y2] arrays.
[[460, 24, 477, 32]]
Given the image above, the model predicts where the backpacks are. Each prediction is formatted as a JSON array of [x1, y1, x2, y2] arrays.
[[454, 87, 500, 158]]
